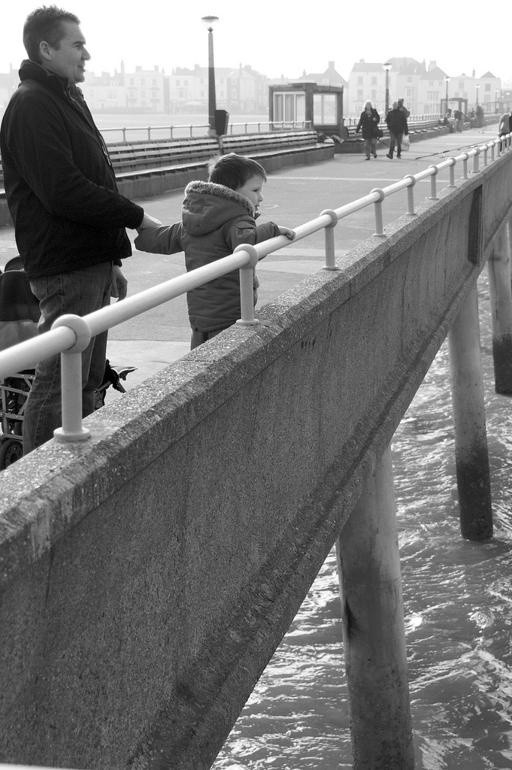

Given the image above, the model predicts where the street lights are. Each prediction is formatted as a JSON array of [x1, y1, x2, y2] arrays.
[[201, 15, 222, 138], [474, 84, 480, 107], [494, 87, 499, 114], [443, 75, 450, 118], [382, 61, 391, 117]]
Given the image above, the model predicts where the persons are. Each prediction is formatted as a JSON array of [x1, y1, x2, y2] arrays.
[[440, 106, 484, 132], [134, 153, 297, 350], [393, 98, 411, 151], [383, 97, 408, 160], [357, 101, 382, 160], [1, 1, 163, 457], [495, 113, 512, 152]]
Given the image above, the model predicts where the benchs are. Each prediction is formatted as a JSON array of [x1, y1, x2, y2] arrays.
[[345, 119, 447, 153], [105, 130, 338, 199]]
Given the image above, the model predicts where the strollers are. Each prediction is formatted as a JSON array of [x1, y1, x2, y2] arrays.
[[0, 253, 135, 468]]
